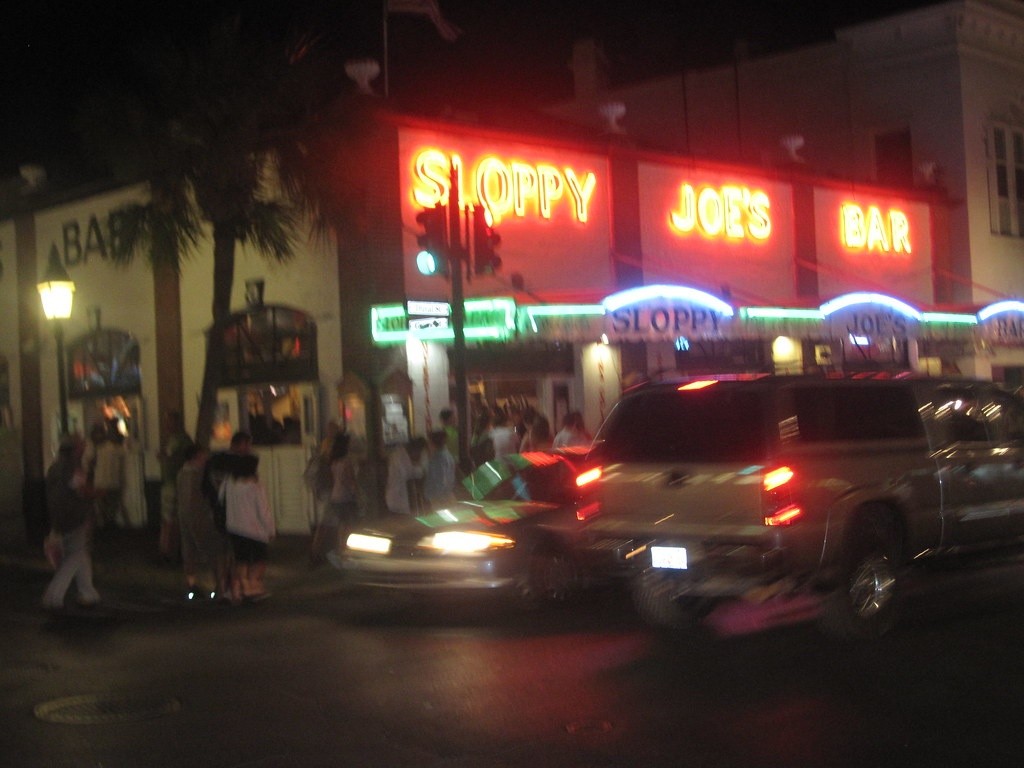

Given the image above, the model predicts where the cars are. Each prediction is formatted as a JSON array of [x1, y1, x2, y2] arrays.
[[329, 445, 591, 611]]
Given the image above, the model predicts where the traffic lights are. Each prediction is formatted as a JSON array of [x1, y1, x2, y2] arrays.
[[416, 203, 450, 280], [474, 210, 501, 275]]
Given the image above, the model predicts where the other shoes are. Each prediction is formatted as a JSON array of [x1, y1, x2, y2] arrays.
[[250, 588, 273, 601]]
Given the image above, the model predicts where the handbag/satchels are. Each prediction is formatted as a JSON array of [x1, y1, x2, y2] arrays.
[[43, 531, 65, 574], [54, 503, 89, 536], [159, 507, 180, 553], [214, 472, 229, 536]]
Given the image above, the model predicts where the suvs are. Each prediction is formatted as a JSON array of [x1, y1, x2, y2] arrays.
[[534, 368, 1024, 634]]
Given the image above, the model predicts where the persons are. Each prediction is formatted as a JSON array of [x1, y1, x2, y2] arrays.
[[79, 404, 196, 561], [39, 434, 105, 613], [302, 405, 593, 572], [174, 444, 229, 601], [218, 452, 277, 600], [204, 429, 258, 580]]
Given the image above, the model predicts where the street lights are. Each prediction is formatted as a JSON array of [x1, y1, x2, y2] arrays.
[[32, 241, 78, 436]]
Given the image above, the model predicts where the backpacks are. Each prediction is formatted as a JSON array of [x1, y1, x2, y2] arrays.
[[304, 450, 331, 500]]
